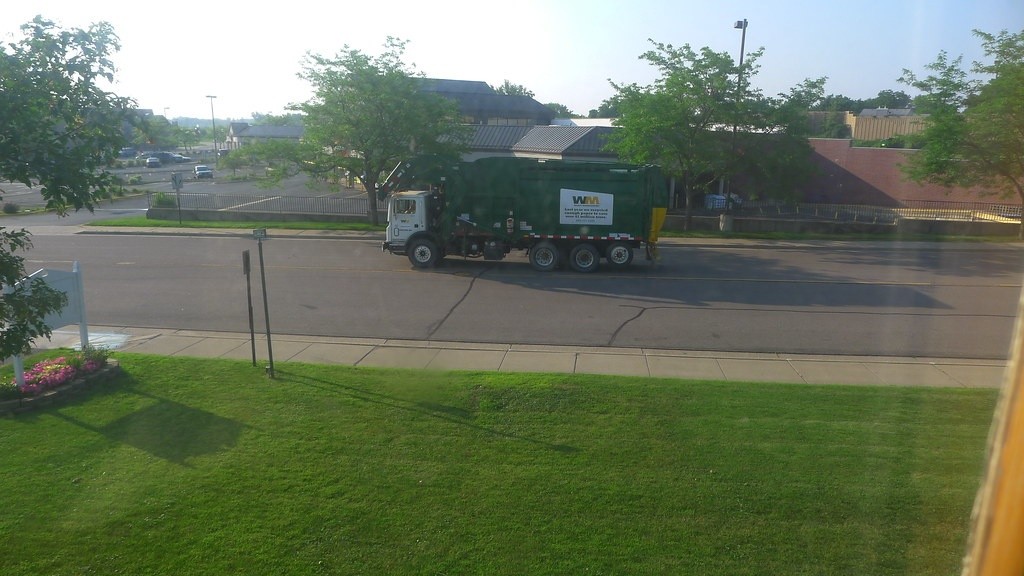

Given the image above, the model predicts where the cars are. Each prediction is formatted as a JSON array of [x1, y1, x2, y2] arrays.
[[146, 157, 160, 168], [193, 164, 213, 180], [136, 151, 192, 163]]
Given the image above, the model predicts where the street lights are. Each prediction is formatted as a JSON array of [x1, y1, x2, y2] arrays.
[[253, 228, 275, 380], [724, 19, 749, 213], [206, 96, 218, 167]]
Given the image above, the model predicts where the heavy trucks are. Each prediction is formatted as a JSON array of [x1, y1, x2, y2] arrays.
[[382, 161, 657, 275]]
